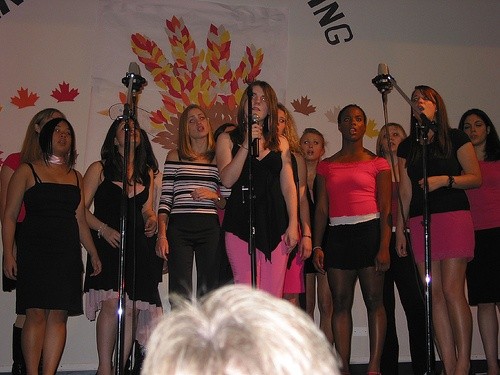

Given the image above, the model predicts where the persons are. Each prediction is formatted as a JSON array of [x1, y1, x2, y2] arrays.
[[221, 79, 297, 298], [132, 129, 169, 375], [312, 104, 392, 375], [213, 123, 238, 289], [155, 104, 225, 310], [395, 85, 483, 375], [277, 102, 312, 308], [140, 281, 343, 374], [1, 117, 103, 375], [83, 114, 157, 375], [0, 108, 65, 375], [459, 108, 500, 375], [299, 128, 334, 348], [376, 123, 438, 375]]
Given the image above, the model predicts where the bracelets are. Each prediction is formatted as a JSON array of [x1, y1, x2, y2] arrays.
[[312, 246, 322, 251], [156, 235, 168, 240], [240, 143, 249, 151], [97, 222, 108, 238], [442, 175, 454, 189], [302, 235, 312, 239]]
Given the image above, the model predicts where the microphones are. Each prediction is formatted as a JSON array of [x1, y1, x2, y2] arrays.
[[378, 63, 388, 94], [252, 113, 261, 157], [129, 62, 139, 103]]
